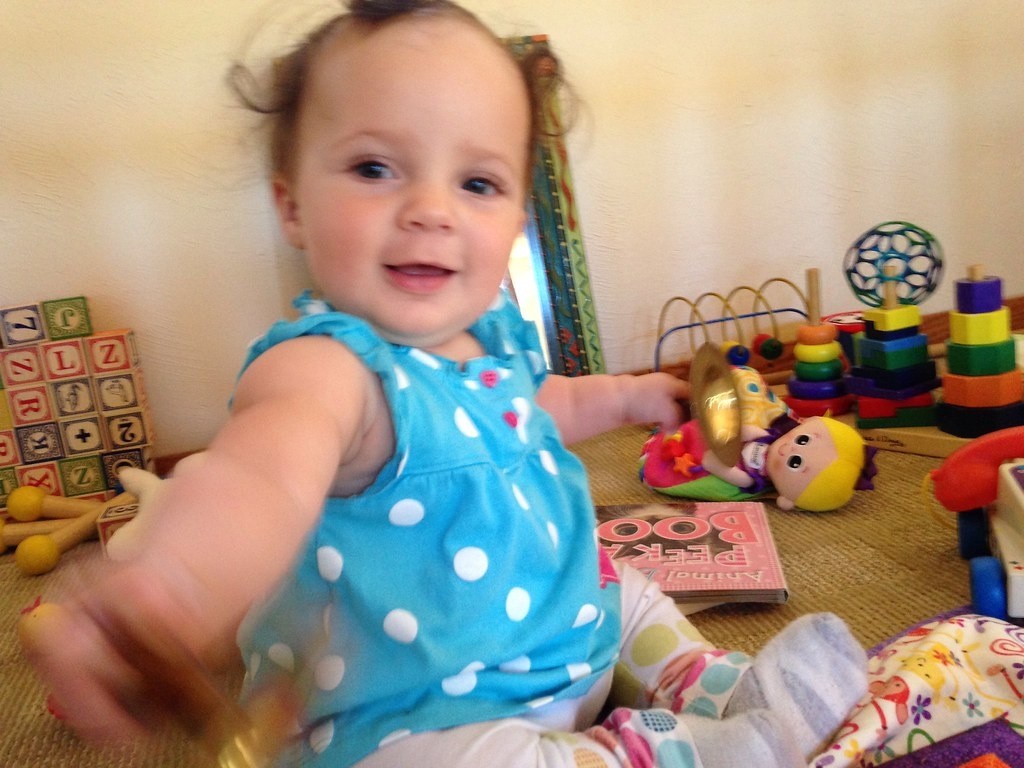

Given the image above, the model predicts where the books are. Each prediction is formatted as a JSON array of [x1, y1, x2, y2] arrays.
[[592, 502, 788, 616]]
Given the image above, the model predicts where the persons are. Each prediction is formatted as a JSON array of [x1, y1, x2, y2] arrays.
[[25, 0, 871, 768]]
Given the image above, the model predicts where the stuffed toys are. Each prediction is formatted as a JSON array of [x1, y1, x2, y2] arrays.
[[636, 366, 878, 511]]
